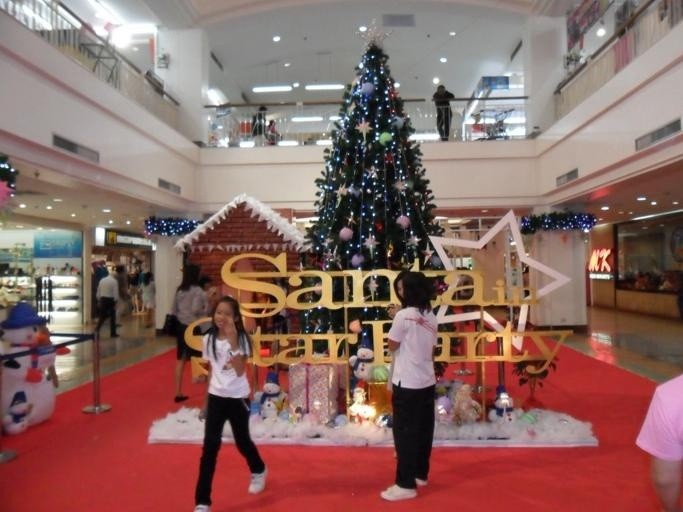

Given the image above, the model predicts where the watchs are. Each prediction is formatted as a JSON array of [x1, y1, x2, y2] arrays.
[[227, 349, 238, 357]]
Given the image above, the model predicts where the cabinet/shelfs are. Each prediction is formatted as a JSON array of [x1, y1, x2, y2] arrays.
[[32, 274, 81, 315]]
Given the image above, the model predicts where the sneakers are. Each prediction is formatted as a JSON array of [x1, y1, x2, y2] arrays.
[[248, 464, 267, 495], [379, 484, 417, 502], [193, 503, 209, 512], [110, 333, 120, 337], [415, 477, 428, 486]]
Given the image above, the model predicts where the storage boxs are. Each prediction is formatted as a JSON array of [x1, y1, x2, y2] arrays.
[[366, 378, 390, 423], [285, 361, 346, 428]]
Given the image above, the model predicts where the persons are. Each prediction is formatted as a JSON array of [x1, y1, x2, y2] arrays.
[[633, 373, 682, 512], [385, 272, 411, 321], [113, 265, 130, 327], [266, 120, 279, 146], [95, 271, 120, 338], [380, 270, 439, 503], [192, 295, 269, 511], [137, 272, 155, 326], [251, 106, 267, 148], [430, 85, 456, 141]]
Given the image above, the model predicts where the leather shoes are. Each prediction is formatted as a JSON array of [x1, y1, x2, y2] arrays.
[[175, 394, 189, 402]]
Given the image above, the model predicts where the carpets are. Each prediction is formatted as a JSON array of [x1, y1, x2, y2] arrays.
[[1, 317, 681, 512]]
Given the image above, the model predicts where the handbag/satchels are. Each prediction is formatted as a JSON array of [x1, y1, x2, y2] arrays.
[[162, 313, 176, 337]]
